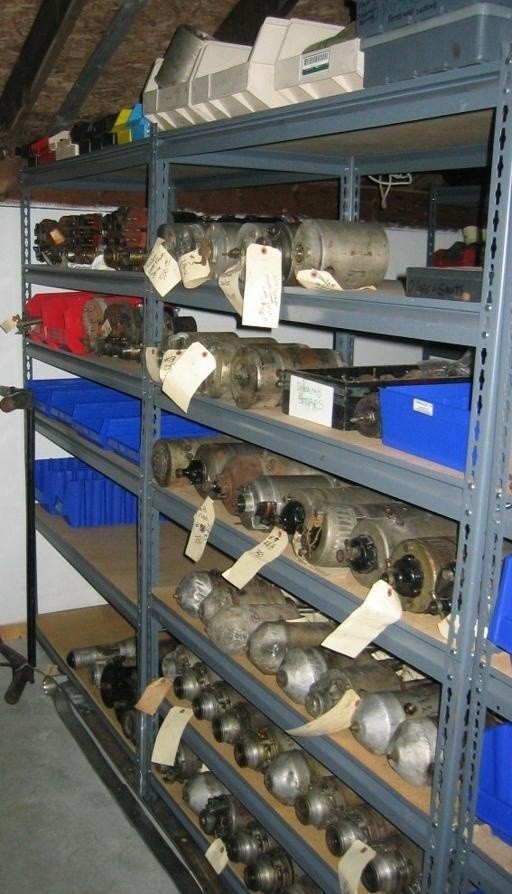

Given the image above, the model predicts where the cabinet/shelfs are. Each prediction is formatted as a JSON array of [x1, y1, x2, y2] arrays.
[[16, 43, 510, 894]]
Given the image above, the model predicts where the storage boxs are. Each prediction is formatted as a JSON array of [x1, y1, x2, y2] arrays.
[[25, 378, 104, 415], [49, 387, 140, 427], [468, 722, 511, 843], [73, 401, 168, 446], [489, 556, 511, 656], [32, 457, 168, 526], [31, 103, 153, 165], [404, 267, 494, 304], [280, 363, 469, 431], [103, 415, 221, 465], [378, 381, 482, 476]]
